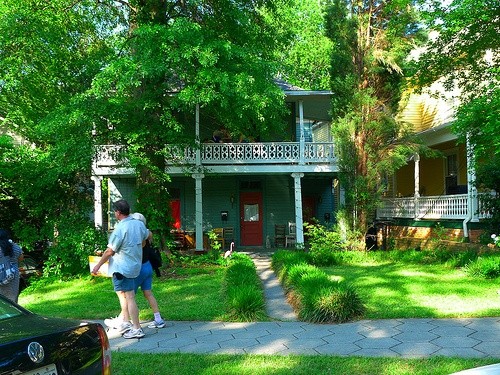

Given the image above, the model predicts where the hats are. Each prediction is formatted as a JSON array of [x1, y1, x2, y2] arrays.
[[129, 212, 146, 224]]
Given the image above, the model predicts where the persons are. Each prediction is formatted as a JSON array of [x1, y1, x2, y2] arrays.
[[0, 230, 24, 303], [92, 200, 166, 339]]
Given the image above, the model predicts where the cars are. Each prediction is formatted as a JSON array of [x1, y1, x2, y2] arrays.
[[0, 291, 114, 375], [17, 253, 43, 288]]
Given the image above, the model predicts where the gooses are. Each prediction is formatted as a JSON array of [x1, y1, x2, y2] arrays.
[[224, 242, 234, 258]]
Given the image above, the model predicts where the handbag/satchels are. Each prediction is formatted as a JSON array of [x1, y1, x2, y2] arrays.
[[146, 238, 162, 277], [0, 248, 16, 286]]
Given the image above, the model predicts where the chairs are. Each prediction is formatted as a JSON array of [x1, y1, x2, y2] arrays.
[[211, 227, 234, 250], [273, 222, 296, 248]]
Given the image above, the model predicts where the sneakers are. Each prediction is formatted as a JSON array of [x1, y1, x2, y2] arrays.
[[104, 318, 123, 326], [117, 320, 133, 333], [147, 319, 166, 328], [123, 326, 145, 338]]
